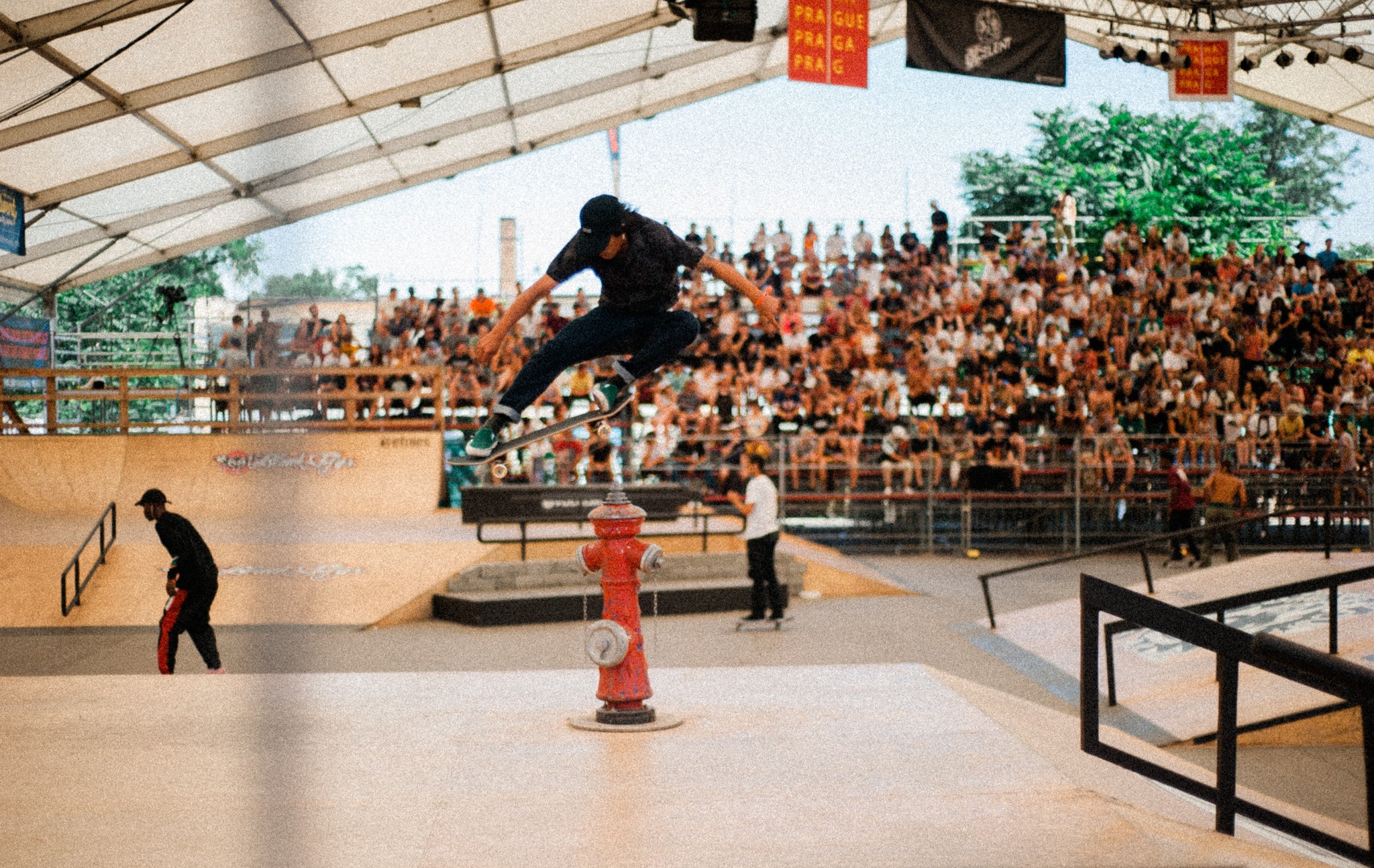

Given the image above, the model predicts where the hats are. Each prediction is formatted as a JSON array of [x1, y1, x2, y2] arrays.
[[232, 315, 244, 322], [576, 195, 622, 254], [134, 488, 171, 505], [892, 426, 908, 439]]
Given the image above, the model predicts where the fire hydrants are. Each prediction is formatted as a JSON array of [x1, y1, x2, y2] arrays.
[[571, 480, 685, 733]]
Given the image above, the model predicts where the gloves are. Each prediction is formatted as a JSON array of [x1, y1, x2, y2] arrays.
[[166, 581, 176, 597]]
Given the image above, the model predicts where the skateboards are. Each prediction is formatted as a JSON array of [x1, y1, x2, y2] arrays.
[[1161, 556, 1205, 568], [730, 618, 792, 634], [446, 393, 634, 479]]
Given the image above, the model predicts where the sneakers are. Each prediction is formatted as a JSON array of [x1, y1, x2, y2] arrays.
[[593, 382, 618, 413], [466, 428, 501, 458]]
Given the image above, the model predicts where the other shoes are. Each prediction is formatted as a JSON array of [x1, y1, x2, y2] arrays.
[[741, 613, 765, 619], [768, 613, 784, 619]]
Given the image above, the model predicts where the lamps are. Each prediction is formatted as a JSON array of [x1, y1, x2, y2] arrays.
[[672, 0, 757, 42], [1342, 46, 1363, 62], [1304, 48, 1329, 66], [1098, 29, 1192, 70], [1237, 52, 1262, 73], [1274, 49, 1294, 69]]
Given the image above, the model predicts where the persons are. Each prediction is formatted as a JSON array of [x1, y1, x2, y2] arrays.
[[1156, 453, 1202, 562], [210, 198, 1371, 508], [1200, 461, 1247, 565], [134, 487, 231, 675], [81, 382, 125, 435], [466, 195, 780, 457], [728, 452, 789, 621]]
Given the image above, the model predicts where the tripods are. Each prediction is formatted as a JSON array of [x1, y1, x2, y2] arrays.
[[136, 309, 188, 389]]
